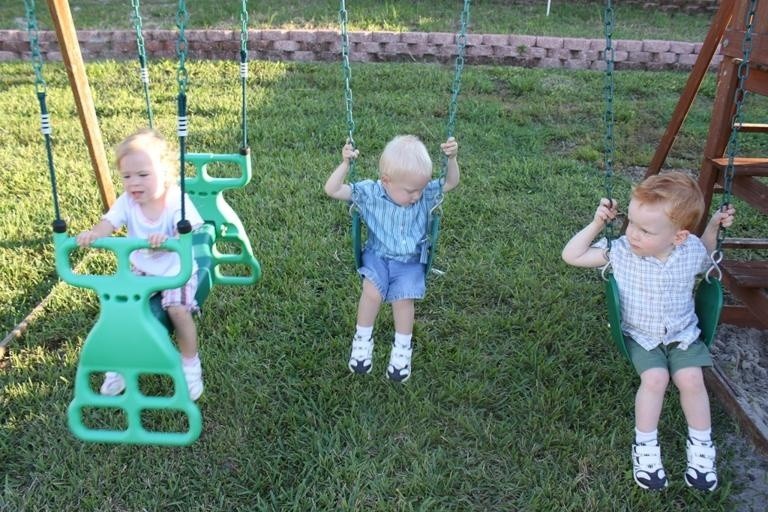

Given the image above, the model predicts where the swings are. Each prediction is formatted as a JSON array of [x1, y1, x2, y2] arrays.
[[600, 0, 758, 365], [340, 0, 469, 283], [25, 0, 262, 445]]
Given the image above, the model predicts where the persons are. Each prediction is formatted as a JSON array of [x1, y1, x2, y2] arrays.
[[76, 130, 204, 401], [561, 171, 736, 490], [324, 135, 460, 381]]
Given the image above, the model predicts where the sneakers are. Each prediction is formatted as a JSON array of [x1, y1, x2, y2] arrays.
[[683, 435, 719, 494], [181, 358, 205, 402], [99, 371, 125, 396], [385, 343, 415, 383], [348, 334, 375, 375], [631, 442, 670, 492]]
